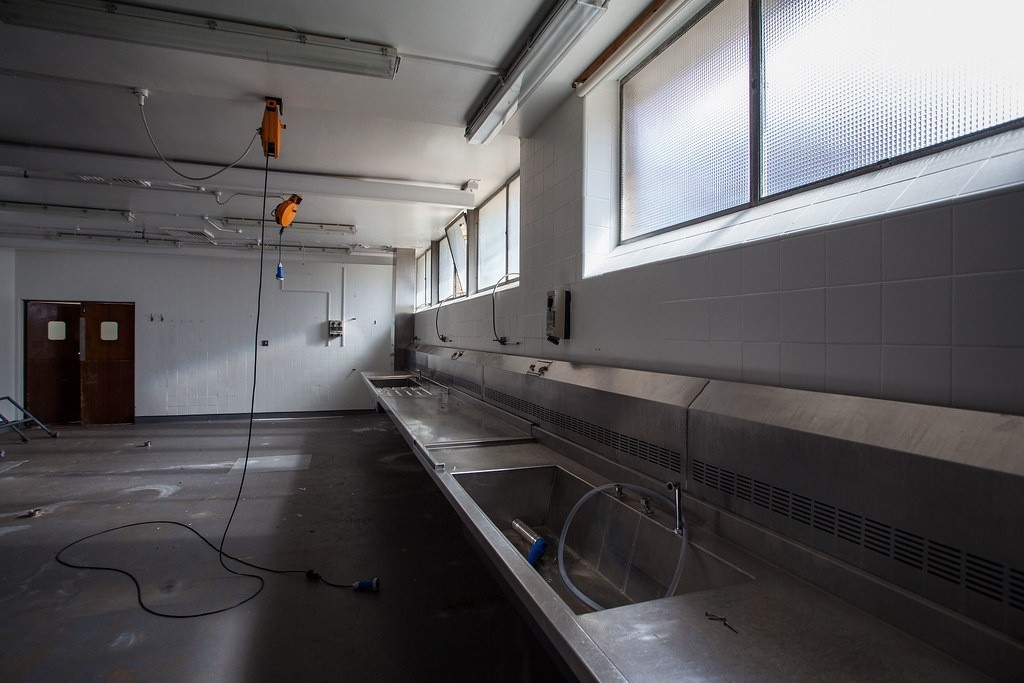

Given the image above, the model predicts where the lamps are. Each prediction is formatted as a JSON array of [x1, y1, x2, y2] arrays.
[[572, 0, 693, 95], [463, 0, 611, 145], [0, 201, 136, 226], [218, 217, 358, 237], [0, 1, 404, 80]]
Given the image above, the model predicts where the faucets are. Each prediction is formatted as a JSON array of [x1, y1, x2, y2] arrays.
[[666, 480, 687, 535], [416, 370, 422, 380]]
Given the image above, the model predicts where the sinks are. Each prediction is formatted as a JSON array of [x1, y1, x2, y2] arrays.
[[367, 378, 422, 389], [448, 462, 756, 620]]
